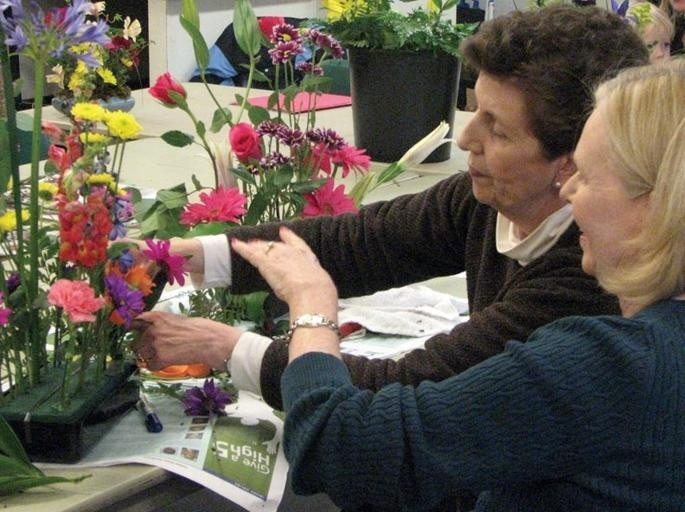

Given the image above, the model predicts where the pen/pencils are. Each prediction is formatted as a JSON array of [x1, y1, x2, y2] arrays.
[[139, 392, 163, 433]]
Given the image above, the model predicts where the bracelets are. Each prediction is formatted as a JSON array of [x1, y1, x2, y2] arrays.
[[223, 352, 231, 377]]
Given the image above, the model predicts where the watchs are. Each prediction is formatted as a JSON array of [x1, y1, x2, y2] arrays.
[[286, 313, 341, 351]]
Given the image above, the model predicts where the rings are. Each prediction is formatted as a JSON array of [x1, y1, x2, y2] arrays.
[[265, 241, 275, 254]]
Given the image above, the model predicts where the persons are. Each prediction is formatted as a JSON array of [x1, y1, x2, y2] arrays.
[[624, 1, 685, 59], [106, 0, 651, 412], [231, 55, 684, 512]]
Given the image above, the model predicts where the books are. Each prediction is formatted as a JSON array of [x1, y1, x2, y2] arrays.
[[339, 322, 367, 342]]
